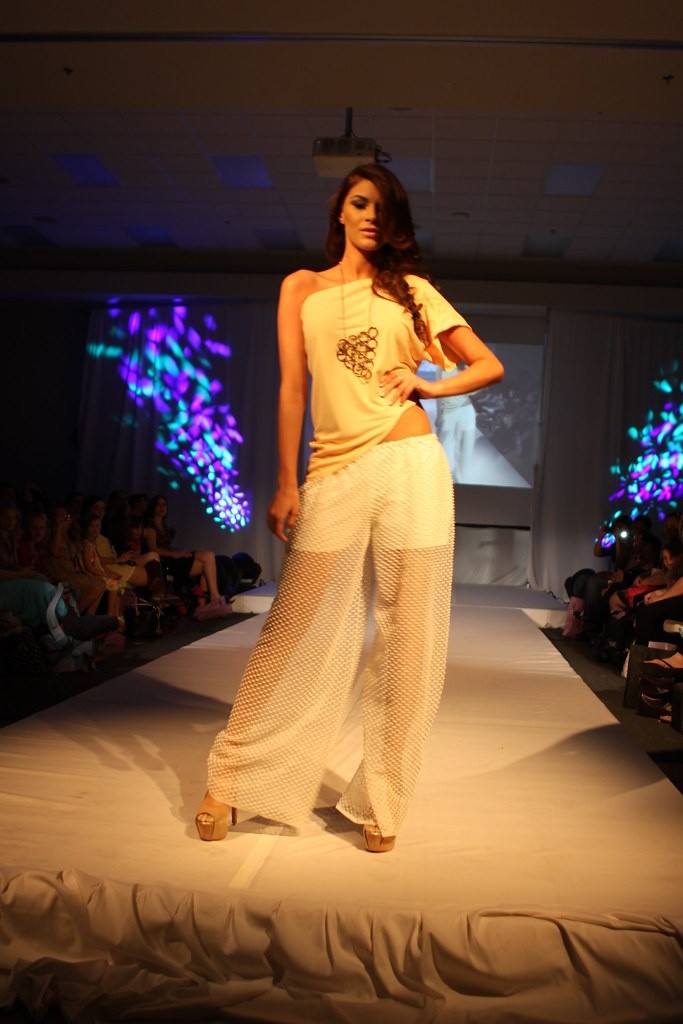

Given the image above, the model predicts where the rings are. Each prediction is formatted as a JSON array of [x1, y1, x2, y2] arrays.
[[398, 377, 402, 381]]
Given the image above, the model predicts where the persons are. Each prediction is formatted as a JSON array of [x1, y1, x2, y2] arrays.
[[476, 385, 537, 456], [434, 367, 476, 482], [557, 512, 683, 722], [0, 482, 235, 661], [196, 164, 505, 851]]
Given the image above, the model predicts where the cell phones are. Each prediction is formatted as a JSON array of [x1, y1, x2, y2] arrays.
[[603, 526, 617, 533], [619, 530, 635, 539], [60, 513, 73, 523]]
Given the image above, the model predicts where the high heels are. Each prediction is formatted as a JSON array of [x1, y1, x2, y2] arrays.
[[363, 825, 395, 852], [195, 789, 238, 842]]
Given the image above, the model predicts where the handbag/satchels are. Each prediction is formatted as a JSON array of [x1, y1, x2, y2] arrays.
[[0, 626, 49, 677], [124, 596, 163, 640]]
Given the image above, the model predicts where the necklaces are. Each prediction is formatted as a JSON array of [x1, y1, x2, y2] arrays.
[[336, 262, 378, 385]]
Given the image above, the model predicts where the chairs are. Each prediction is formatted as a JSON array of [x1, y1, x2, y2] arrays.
[[214, 554, 237, 598], [233, 552, 263, 594]]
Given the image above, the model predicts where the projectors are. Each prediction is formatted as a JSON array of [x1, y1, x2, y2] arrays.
[[311, 136, 379, 177]]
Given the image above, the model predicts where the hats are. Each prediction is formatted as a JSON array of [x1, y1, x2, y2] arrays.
[[613, 516, 632, 529]]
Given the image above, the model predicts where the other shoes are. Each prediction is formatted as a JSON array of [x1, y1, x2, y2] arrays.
[[598, 650, 625, 663], [201, 592, 211, 606], [225, 595, 235, 604], [80, 652, 100, 676], [636, 693, 674, 716], [590, 637, 602, 649], [573, 603, 596, 623], [640, 651, 682, 675]]
